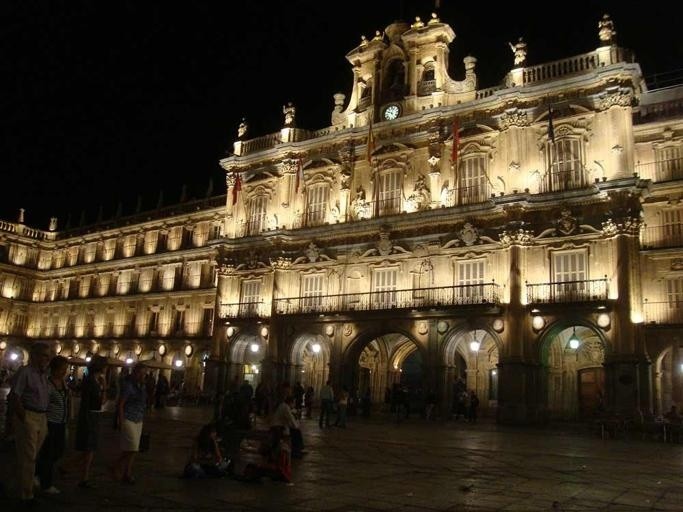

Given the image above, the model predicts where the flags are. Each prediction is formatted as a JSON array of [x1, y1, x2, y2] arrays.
[[451, 121, 460, 161], [295, 159, 299, 192], [232, 172, 241, 205], [369, 122, 372, 161], [548, 102, 554, 143]]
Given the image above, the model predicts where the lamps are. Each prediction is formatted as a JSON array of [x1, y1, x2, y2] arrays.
[[419, 62, 605, 110], [217, 171, 638, 241], [85, 350, 182, 368], [250, 335, 257, 351], [224, 295, 601, 318], [312, 336, 319, 352], [469, 329, 479, 351], [569, 328, 578, 350], [306, 120, 357, 141]]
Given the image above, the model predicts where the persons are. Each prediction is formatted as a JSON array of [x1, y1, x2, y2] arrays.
[[283, 102, 296, 127], [664, 406, 682, 424], [597, 12, 617, 46], [509, 37, 529, 65]]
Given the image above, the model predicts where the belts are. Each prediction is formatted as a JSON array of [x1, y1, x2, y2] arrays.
[[26, 407, 46, 413]]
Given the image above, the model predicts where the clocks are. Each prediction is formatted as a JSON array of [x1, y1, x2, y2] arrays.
[[381, 103, 402, 122]]
